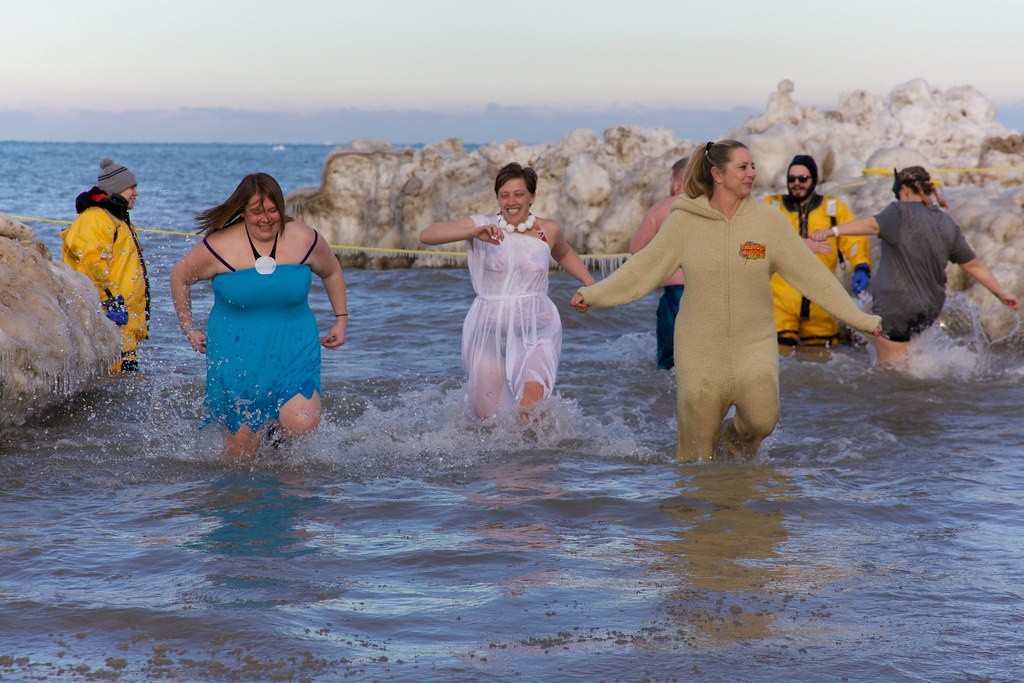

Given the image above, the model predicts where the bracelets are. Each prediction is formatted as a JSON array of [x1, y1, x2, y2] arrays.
[[335, 313, 349, 317]]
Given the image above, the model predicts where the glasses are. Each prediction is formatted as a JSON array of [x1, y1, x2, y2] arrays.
[[788, 175, 812, 183]]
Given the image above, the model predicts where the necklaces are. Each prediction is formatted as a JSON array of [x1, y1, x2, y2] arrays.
[[496, 209, 536, 234]]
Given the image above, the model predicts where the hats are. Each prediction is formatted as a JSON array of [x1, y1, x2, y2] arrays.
[[787, 155, 818, 202], [97, 159, 138, 196]]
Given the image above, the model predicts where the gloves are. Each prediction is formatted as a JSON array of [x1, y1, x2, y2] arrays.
[[851, 263, 870, 295], [103, 295, 128, 325]]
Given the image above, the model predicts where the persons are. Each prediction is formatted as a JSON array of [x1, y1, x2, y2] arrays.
[[419, 160, 600, 438], [758, 153, 873, 356], [60, 156, 151, 380], [629, 153, 702, 368], [570, 137, 885, 466], [810, 166, 1021, 375], [170, 173, 350, 467]]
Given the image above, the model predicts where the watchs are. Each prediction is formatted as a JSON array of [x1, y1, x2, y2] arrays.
[[828, 225, 840, 239]]
